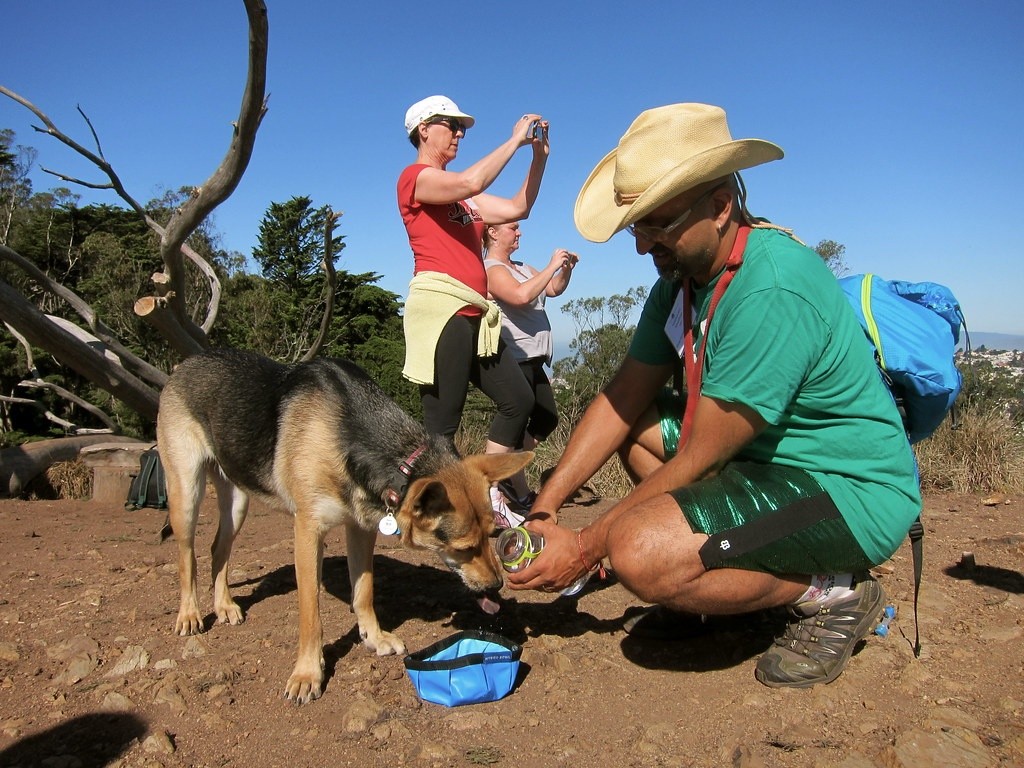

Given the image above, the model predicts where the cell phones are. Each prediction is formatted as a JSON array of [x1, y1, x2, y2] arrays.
[[565, 255, 573, 265], [534, 122, 543, 142]]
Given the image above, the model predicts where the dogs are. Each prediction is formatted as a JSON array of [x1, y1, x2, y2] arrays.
[[156, 347, 537, 705]]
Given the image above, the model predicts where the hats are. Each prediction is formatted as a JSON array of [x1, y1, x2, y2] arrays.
[[574, 102, 785, 243], [405, 95, 475, 134]]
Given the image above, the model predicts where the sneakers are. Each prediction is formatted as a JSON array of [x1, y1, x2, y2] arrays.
[[519, 491, 538, 511], [499, 478, 520, 504], [754, 568, 887, 688]]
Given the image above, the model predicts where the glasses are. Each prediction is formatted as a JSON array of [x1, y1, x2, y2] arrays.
[[426, 117, 466, 139], [625, 182, 728, 242]]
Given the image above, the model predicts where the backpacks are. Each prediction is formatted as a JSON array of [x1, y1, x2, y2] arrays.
[[833, 275, 970, 446], [124, 444, 170, 511]]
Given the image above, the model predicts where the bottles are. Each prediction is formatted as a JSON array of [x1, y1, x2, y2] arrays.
[[495, 529, 588, 595]]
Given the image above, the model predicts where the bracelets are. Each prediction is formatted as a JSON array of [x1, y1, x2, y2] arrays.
[[578, 529, 607, 580]]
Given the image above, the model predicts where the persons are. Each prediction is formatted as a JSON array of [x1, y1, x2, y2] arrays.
[[483, 222, 580, 504], [397, 92, 551, 511], [503, 101, 921, 689]]
